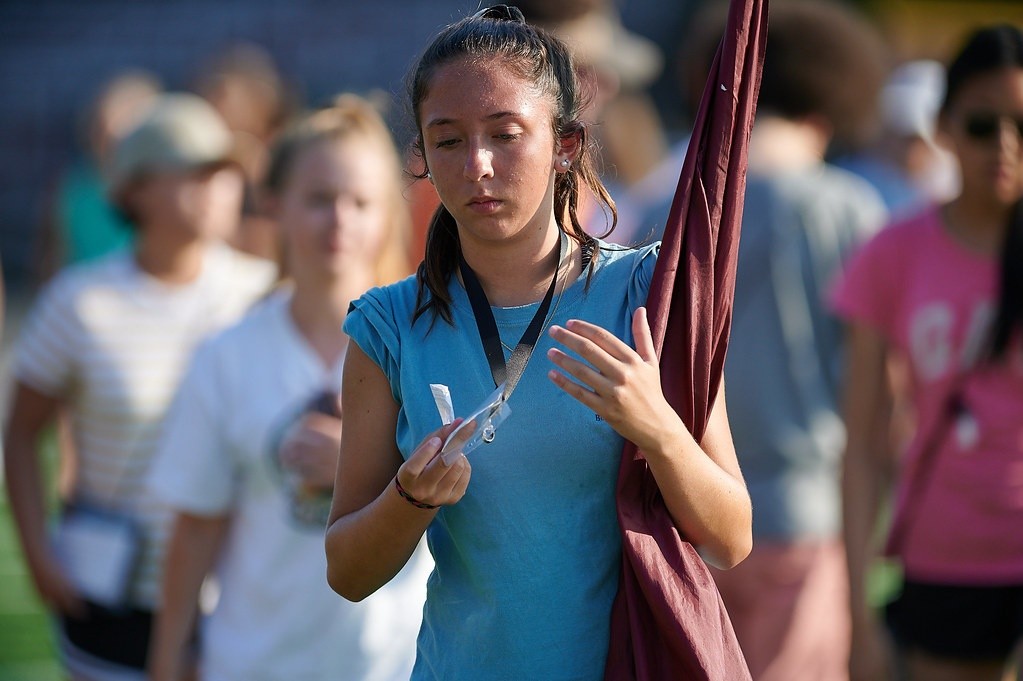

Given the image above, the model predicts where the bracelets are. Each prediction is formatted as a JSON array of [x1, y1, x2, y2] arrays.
[[395, 478, 443, 509]]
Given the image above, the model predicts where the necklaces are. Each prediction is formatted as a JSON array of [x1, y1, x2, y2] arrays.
[[501, 237, 573, 363]]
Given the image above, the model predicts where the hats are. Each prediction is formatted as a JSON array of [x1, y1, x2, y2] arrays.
[[108, 90, 234, 194]]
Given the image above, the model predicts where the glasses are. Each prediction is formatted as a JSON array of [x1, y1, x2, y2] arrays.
[[947, 103, 1023, 148]]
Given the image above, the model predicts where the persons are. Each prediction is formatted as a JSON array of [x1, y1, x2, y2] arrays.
[[0, 92, 284, 679], [830, 23, 1023, 681], [33, 79, 164, 508], [627, 11, 893, 681], [326, 5, 754, 680], [147, 95, 432, 681], [497, 1, 962, 240], [195, 45, 287, 269]]
